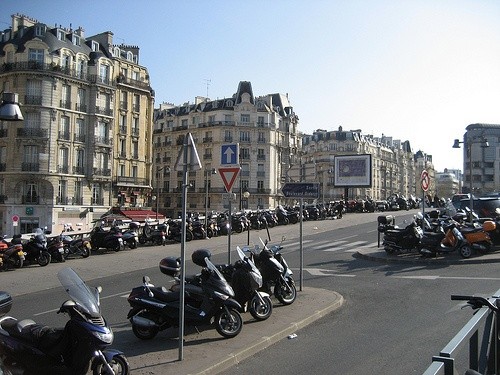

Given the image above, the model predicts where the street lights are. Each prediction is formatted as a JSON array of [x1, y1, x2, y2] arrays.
[[452, 139, 490, 223], [205, 168, 216, 239], [156, 165, 171, 219]]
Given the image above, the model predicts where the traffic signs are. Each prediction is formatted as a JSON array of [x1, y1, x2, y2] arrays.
[[281, 182, 319, 199]]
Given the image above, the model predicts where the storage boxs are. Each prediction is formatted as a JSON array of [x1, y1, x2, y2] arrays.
[[0, 290, 12, 313], [130, 221, 140, 229], [159, 256, 181, 277], [378, 214, 393, 225], [483, 221, 496, 231], [192, 248, 211, 268], [159, 224, 168, 230]]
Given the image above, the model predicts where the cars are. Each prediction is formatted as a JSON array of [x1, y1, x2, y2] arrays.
[[440, 197, 500, 218], [452, 193, 474, 202]]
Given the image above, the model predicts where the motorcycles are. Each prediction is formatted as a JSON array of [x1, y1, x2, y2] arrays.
[[159, 247, 273, 321], [126, 257, 243, 341], [214, 235, 295, 299], [377, 207, 500, 258], [163, 201, 347, 243], [1, 267, 130, 375], [192, 238, 297, 305], [348, 195, 429, 213], [0, 218, 172, 271]]
